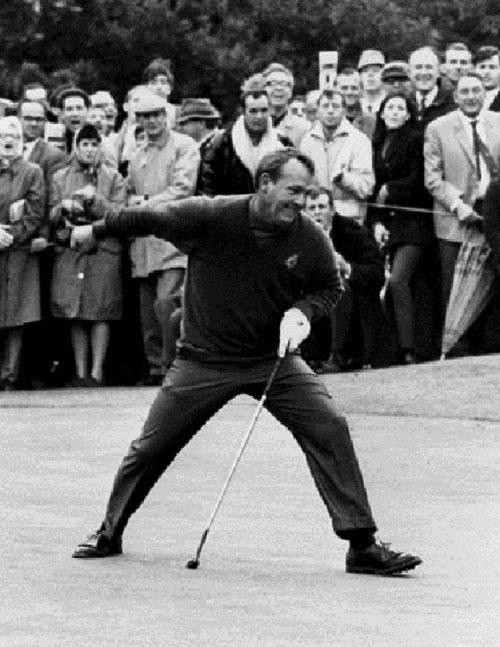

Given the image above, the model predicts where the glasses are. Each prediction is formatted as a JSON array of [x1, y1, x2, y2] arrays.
[[264, 79, 291, 88]]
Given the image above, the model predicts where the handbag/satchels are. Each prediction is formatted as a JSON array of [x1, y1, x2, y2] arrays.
[[9, 199, 26, 222], [48, 195, 98, 256]]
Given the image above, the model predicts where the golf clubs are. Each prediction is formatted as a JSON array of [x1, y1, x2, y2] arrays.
[[185, 357, 282, 569]]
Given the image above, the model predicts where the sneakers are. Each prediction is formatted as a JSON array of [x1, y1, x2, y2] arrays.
[[72, 534, 122, 558], [346, 541, 423, 574]]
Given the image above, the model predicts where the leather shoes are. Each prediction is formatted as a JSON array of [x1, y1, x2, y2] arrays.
[[77, 375, 107, 387], [68, 375, 85, 388], [403, 349, 420, 364], [136, 375, 164, 386], [322, 361, 344, 373]]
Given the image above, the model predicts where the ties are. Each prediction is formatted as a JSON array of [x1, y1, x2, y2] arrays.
[[471, 121, 481, 180], [368, 105, 372, 112]]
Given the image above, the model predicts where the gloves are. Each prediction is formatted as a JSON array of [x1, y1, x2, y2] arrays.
[[70, 225, 97, 254], [278, 309, 311, 358]]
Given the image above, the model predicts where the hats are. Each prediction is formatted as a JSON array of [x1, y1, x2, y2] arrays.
[[133, 95, 167, 114], [381, 61, 408, 81], [175, 98, 220, 122], [358, 50, 384, 69]]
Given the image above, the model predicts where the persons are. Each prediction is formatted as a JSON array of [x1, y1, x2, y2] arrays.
[[143, 62, 179, 131], [174, 96, 225, 146], [59, 88, 93, 152], [379, 59, 410, 94], [422, 72, 500, 355], [473, 44, 500, 111], [16, 98, 71, 253], [441, 43, 472, 87], [302, 185, 385, 374], [0, 114, 48, 391], [356, 48, 387, 112], [69, 150, 421, 576], [297, 88, 375, 227], [481, 173, 499, 278], [88, 106, 109, 147], [195, 89, 294, 197], [335, 66, 376, 140], [405, 44, 456, 130], [47, 123, 126, 388], [287, 88, 320, 123], [21, 82, 58, 122], [125, 94, 203, 386], [89, 90, 119, 147], [367, 90, 435, 365], [261, 62, 313, 150]]
